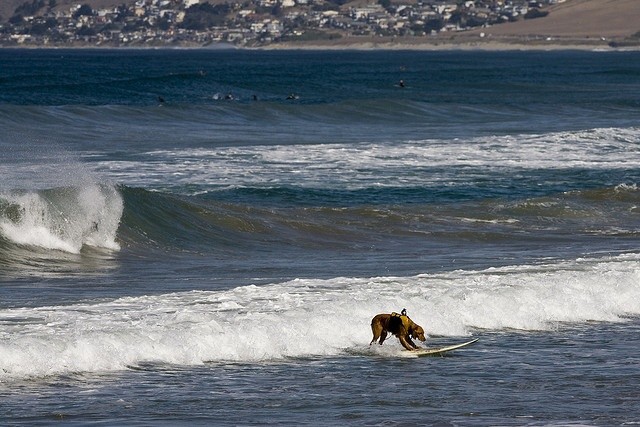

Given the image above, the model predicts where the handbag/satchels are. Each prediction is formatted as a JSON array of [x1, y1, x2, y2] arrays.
[[388, 316, 402, 332]]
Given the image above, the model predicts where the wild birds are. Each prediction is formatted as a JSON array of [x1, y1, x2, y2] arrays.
[[401, 308, 407, 315]]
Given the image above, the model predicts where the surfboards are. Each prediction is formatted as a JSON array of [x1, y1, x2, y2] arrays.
[[397, 337, 479, 356]]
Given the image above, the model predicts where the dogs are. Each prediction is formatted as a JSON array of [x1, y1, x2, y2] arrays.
[[369, 313, 426, 351]]
